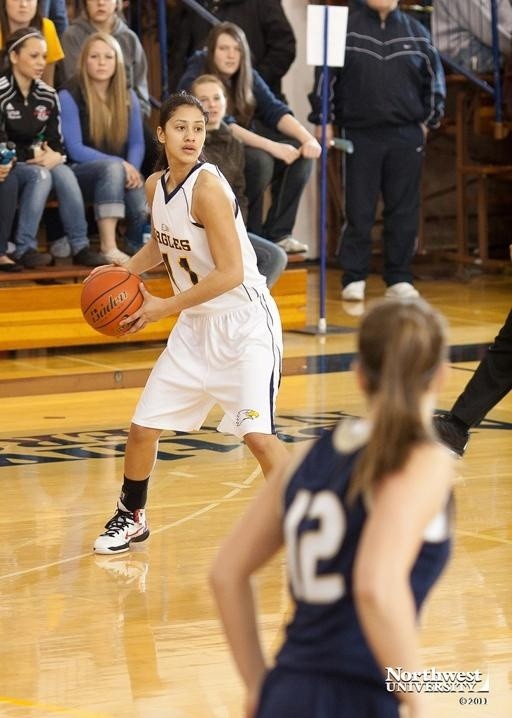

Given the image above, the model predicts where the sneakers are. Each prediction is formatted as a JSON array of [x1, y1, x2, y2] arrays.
[[432, 416, 470, 456], [340, 281, 366, 302], [275, 238, 310, 254], [91, 500, 150, 554], [385, 281, 420, 300], [0, 235, 145, 272]]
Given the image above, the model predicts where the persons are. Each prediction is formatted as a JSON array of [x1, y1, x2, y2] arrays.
[[0, 0, 322, 269], [429, 0, 512, 74], [308, 0, 447, 302], [205, 299, 458, 718], [102, 558, 187, 717], [428, 307, 512, 458], [83, 95, 290, 555]]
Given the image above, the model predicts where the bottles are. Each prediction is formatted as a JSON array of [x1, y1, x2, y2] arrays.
[[0, 140, 16, 164]]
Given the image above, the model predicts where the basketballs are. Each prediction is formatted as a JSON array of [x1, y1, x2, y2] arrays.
[[80, 268, 148, 337]]
